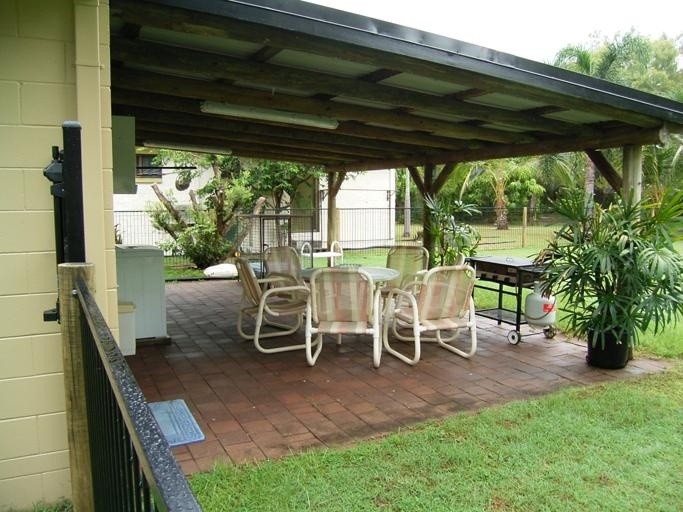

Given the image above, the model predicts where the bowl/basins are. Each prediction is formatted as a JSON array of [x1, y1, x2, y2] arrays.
[[332, 263, 361, 269]]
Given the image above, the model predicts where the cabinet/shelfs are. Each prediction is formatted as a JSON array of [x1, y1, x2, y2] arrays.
[[111, 115, 137, 194], [300, 241, 344, 267]]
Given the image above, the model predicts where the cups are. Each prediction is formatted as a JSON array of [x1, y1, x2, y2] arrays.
[[505, 257, 513, 263]]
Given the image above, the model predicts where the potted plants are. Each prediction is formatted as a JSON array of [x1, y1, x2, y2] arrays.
[[525, 182, 683, 370]]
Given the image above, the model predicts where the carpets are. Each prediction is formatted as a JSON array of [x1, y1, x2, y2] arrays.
[[147, 398, 206, 448]]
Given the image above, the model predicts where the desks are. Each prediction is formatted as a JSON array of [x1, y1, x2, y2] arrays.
[[294, 266, 401, 345]]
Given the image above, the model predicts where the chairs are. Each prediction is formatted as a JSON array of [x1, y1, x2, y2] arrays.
[[262, 246, 310, 332], [391, 252, 466, 343], [374, 244, 430, 329], [383, 263, 478, 366], [233, 257, 319, 354], [305, 267, 384, 369]]
